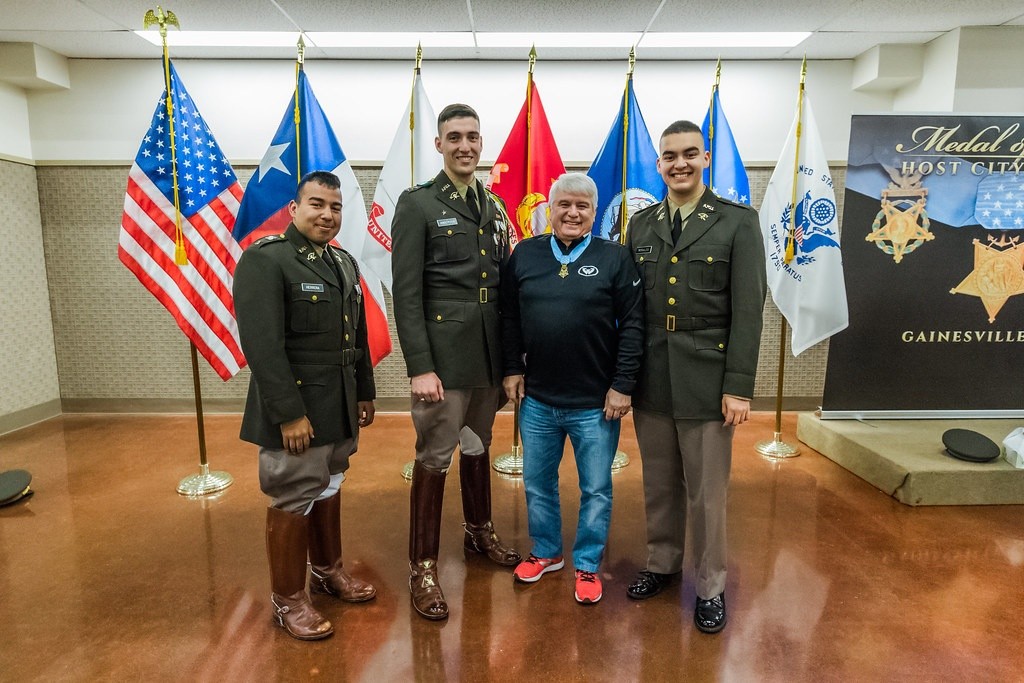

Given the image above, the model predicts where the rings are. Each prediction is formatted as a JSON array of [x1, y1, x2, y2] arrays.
[[421, 398, 425, 401]]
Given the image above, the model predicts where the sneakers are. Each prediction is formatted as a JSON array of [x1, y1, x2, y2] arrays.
[[574, 567, 603, 604], [513, 553, 565, 583]]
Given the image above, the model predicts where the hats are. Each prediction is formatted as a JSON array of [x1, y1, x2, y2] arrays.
[[942, 429, 1000, 462], [0, 469, 34, 507]]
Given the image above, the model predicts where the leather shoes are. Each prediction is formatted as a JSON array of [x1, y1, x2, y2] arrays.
[[694, 591, 726, 634], [626, 570, 682, 600]]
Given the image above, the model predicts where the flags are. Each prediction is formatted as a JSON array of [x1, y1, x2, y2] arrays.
[[119, 51, 249, 380], [758, 88, 849, 357], [360, 75, 444, 296], [487, 80, 566, 257], [231, 71, 392, 368], [701, 89, 750, 207], [587, 78, 668, 244]]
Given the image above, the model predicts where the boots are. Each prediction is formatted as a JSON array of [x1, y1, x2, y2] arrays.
[[407, 458, 449, 620], [307, 486, 377, 602], [409, 606, 449, 683], [264, 506, 335, 640], [458, 448, 523, 565], [460, 557, 514, 683]]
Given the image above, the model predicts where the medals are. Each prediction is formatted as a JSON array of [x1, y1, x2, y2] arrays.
[[948, 170, 1024, 323], [866, 162, 937, 264]]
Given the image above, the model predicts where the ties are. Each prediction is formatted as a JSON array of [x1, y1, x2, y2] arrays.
[[322, 250, 343, 290], [465, 186, 482, 223], [669, 209, 682, 247]]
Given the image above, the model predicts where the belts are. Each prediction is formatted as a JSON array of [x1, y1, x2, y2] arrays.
[[281, 348, 365, 365], [423, 287, 499, 303], [644, 315, 730, 332]]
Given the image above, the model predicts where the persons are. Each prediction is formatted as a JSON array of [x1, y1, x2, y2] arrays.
[[625, 121, 767, 633], [391, 104, 523, 620], [233, 170, 376, 639], [499, 173, 645, 603]]
